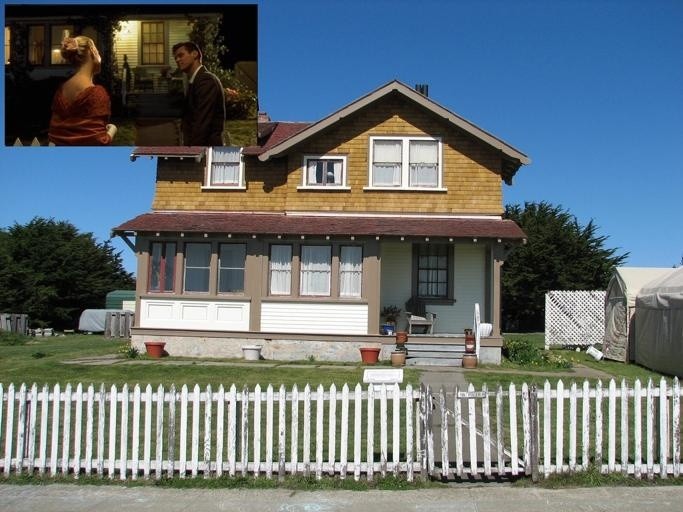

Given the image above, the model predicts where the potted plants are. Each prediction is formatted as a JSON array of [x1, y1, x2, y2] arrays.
[[380, 303, 403, 325]]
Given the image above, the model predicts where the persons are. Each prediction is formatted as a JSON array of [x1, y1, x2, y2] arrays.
[[44, 35, 118, 146], [170, 41, 225, 147]]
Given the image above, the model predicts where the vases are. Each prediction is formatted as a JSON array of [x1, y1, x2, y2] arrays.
[[462, 353, 477, 369], [143, 341, 166, 357], [359, 331, 408, 368]]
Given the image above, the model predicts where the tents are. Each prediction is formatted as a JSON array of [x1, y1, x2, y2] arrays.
[[601, 266, 680, 366], [632, 266, 682, 381]]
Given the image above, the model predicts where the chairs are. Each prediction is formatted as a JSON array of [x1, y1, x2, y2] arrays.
[[404, 295, 434, 334]]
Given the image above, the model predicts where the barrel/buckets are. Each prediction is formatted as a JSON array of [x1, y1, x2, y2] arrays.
[[359, 347, 381, 364], [383, 324, 399, 334], [143, 341, 167, 358]]
